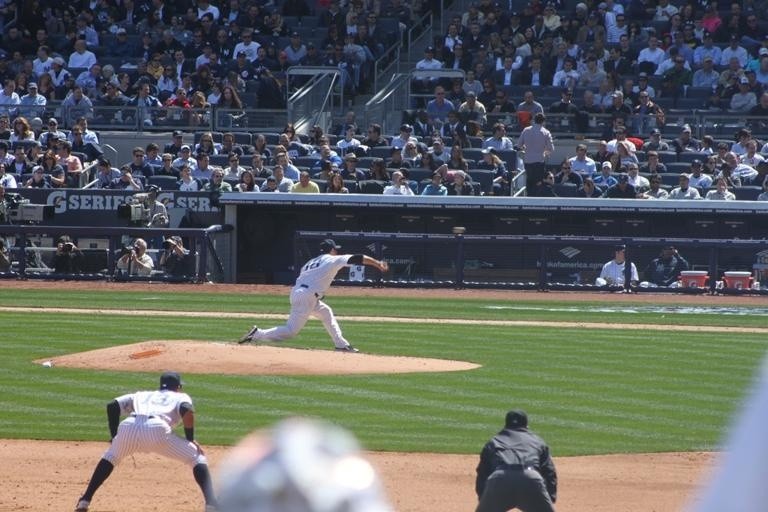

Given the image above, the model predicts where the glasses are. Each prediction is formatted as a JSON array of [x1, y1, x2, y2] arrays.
[[74, 132, 82, 136], [135, 154, 143, 157], [119, 172, 126, 176]]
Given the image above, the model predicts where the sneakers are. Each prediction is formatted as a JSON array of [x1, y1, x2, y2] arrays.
[[74, 497, 89, 512], [335, 345, 360, 353], [239, 325, 257, 343]]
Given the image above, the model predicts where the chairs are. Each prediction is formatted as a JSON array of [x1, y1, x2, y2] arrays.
[[0, 0, 768, 207]]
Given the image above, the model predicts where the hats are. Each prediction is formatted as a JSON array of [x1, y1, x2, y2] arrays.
[[691, 159, 702, 167], [739, 77, 749, 85], [160, 373, 185, 388], [390, 145, 402, 154], [562, 86, 573, 95], [27, 82, 38, 89], [506, 409, 527, 428], [318, 238, 342, 252], [400, 124, 412, 133], [31, 166, 44, 174], [617, 173, 629, 182], [53, 57, 65, 67], [345, 152, 360, 161], [47, 117, 57, 124], [481, 146, 497, 155], [173, 130, 183, 138], [602, 161, 612, 169], [181, 145, 191, 152], [681, 125, 692, 133], [610, 90, 624, 98], [432, 138, 443, 146], [650, 129, 661, 136], [64, 72, 75, 80], [759, 47, 767, 56]]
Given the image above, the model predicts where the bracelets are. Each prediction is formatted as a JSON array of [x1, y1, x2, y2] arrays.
[[185, 428, 194, 442]]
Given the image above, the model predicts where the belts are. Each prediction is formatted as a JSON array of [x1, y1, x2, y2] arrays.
[[493, 463, 537, 471], [301, 284, 318, 298]]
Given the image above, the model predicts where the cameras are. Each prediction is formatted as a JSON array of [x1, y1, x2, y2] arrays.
[[64, 244, 73, 251], [162, 241, 171, 250]]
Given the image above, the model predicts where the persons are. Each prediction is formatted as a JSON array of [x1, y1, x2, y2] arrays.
[[75, 372, 218, 512], [0, 0, 767, 291], [238, 239, 389, 353], [475, 410, 557, 512]]
